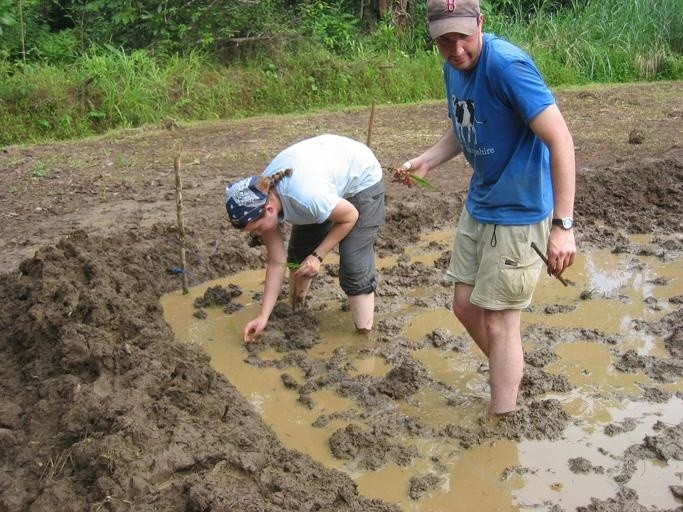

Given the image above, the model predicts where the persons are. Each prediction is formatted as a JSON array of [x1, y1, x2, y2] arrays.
[[224, 134, 385, 344], [392, 0, 577, 413]]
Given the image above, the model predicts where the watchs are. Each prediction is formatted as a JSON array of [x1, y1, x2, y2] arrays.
[[551, 217, 574, 230]]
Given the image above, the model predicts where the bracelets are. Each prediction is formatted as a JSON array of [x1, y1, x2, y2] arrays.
[[310, 252, 323, 263]]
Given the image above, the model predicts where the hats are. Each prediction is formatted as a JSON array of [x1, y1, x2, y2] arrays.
[[427, 0, 480, 41]]
